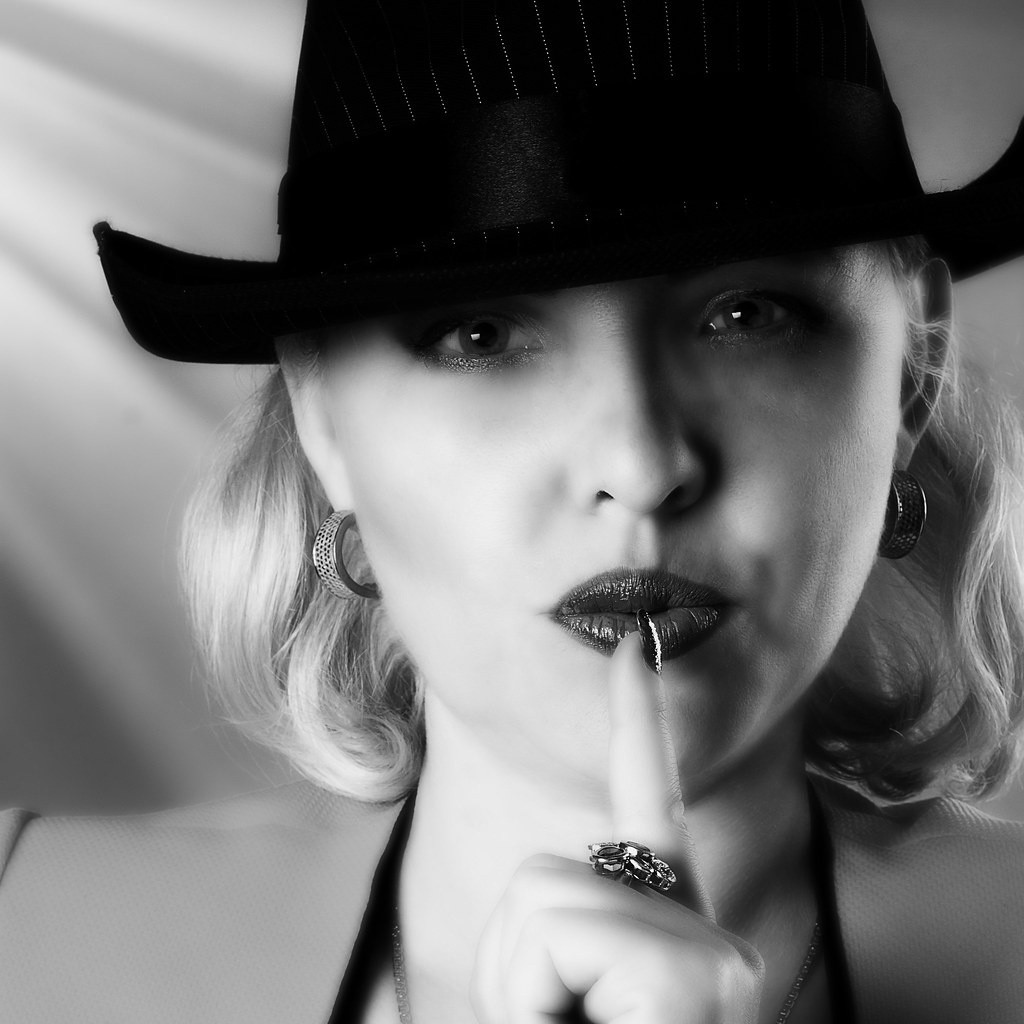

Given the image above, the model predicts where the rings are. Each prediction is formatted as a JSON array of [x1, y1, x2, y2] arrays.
[[587, 841, 676, 891]]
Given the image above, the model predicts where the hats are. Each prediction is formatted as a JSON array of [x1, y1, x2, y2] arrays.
[[86, 1, 1022, 361]]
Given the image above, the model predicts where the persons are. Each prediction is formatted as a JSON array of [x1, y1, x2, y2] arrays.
[[0, 0, 1024, 1024]]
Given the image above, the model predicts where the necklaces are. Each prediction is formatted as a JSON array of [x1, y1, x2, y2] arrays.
[[389, 900, 827, 1023]]
